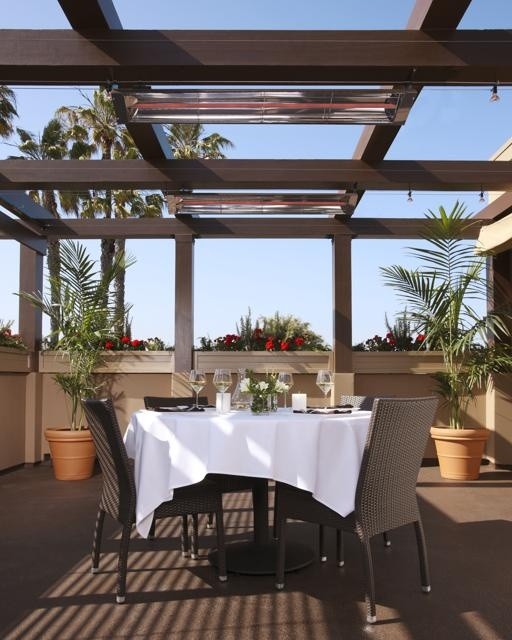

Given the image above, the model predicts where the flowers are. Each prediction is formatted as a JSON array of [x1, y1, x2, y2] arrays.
[[239, 367, 290, 416]]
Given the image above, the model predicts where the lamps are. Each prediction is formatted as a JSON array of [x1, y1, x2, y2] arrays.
[[110, 87, 419, 127], [166, 192, 360, 216]]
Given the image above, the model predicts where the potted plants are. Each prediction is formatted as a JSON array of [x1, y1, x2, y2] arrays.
[[12, 238, 141, 481], [378, 199, 512, 483]]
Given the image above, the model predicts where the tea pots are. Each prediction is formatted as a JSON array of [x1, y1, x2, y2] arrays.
[[232, 367, 254, 411]]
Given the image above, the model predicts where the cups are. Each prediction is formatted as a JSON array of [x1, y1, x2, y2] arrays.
[[291, 394, 306, 412], [278, 371, 294, 411], [213, 368, 233, 415], [315, 369, 334, 412], [215, 393, 230, 414], [188, 369, 208, 411]]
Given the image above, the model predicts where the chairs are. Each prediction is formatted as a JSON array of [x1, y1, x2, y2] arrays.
[[273, 393, 442, 624], [319, 394, 394, 563], [142, 396, 214, 542], [80, 397, 229, 604]]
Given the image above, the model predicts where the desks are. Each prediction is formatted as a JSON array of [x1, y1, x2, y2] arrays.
[[133, 407, 373, 577]]
